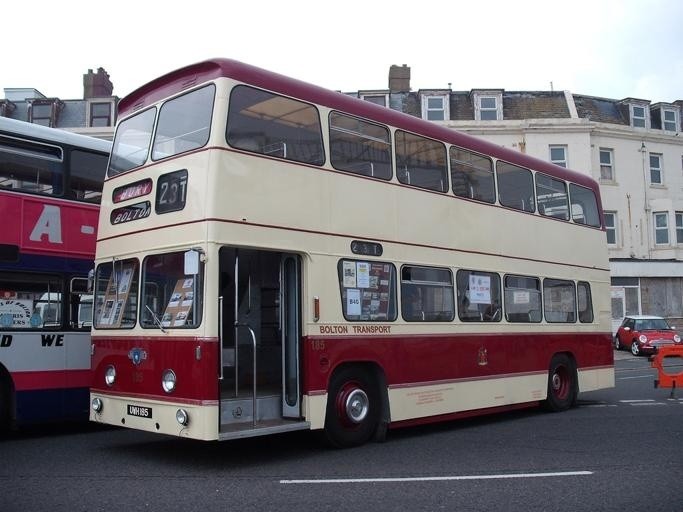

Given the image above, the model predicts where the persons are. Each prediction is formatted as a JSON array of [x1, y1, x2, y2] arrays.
[[485, 299, 499, 321], [458, 295, 470, 320]]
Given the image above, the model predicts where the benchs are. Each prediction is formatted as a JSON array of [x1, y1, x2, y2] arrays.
[[506, 314, 531, 322], [459, 311, 483, 321], [452, 175, 476, 197], [395, 164, 411, 186], [573, 214, 587, 224], [547, 209, 569, 219], [411, 167, 444, 192], [499, 189, 526, 210], [338, 161, 374, 176], [528, 310, 571, 322], [404, 310, 424, 321], [435, 311, 452, 320], [258, 141, 286, 159]]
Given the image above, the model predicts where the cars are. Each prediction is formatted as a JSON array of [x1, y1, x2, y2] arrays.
[[615, 315, 682, 356]]
